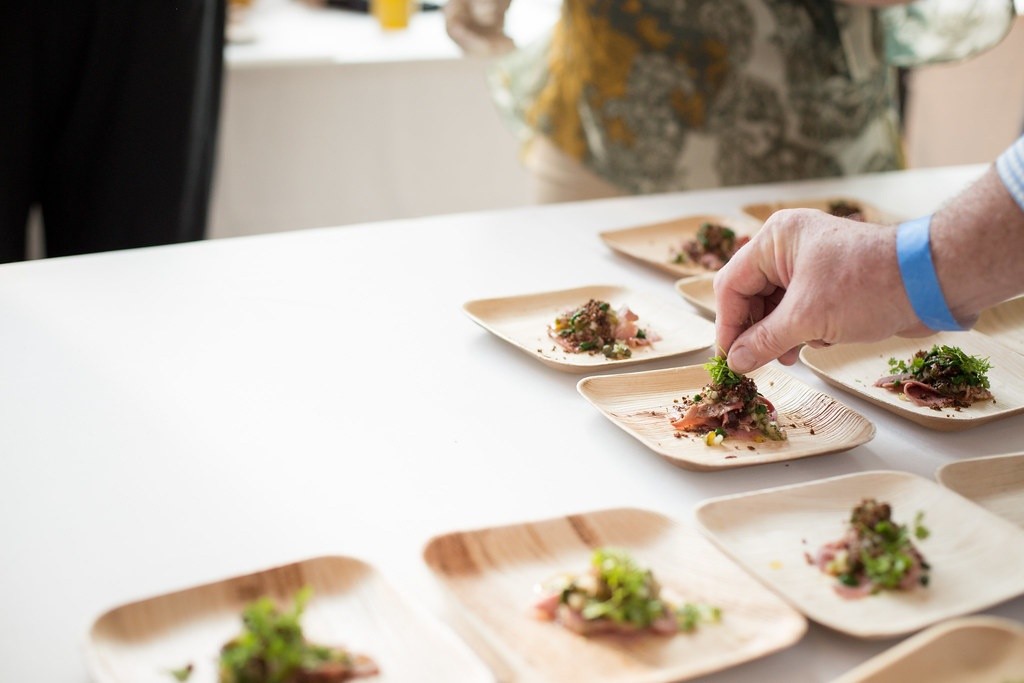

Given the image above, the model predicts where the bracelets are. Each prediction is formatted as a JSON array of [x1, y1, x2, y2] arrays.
[[896, 212, 980, 333]]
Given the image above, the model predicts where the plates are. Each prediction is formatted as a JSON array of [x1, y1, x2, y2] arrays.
[[598, 214, 761, 274], [81, 555, 533, 683], [698, 470, 1024, 641], [746, 198, 902, 226], [576, 363, 875, 472], [677, 276, 716, 322], [464, 286, 716, 375], [424, 507, 809, 683], [937, 453, 1024, 531], [799, 329, 1024, 433], [841, 617, 1024, 683]]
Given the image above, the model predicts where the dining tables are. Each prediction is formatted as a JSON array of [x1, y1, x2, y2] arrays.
[[206, 0, 562, 238], [0, 165, 1024, 683]]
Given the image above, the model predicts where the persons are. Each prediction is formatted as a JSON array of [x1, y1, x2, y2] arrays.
[[710, 133, 1023, 376], [0, 0, 227, 265], [442, 1, 1017, 210]]
